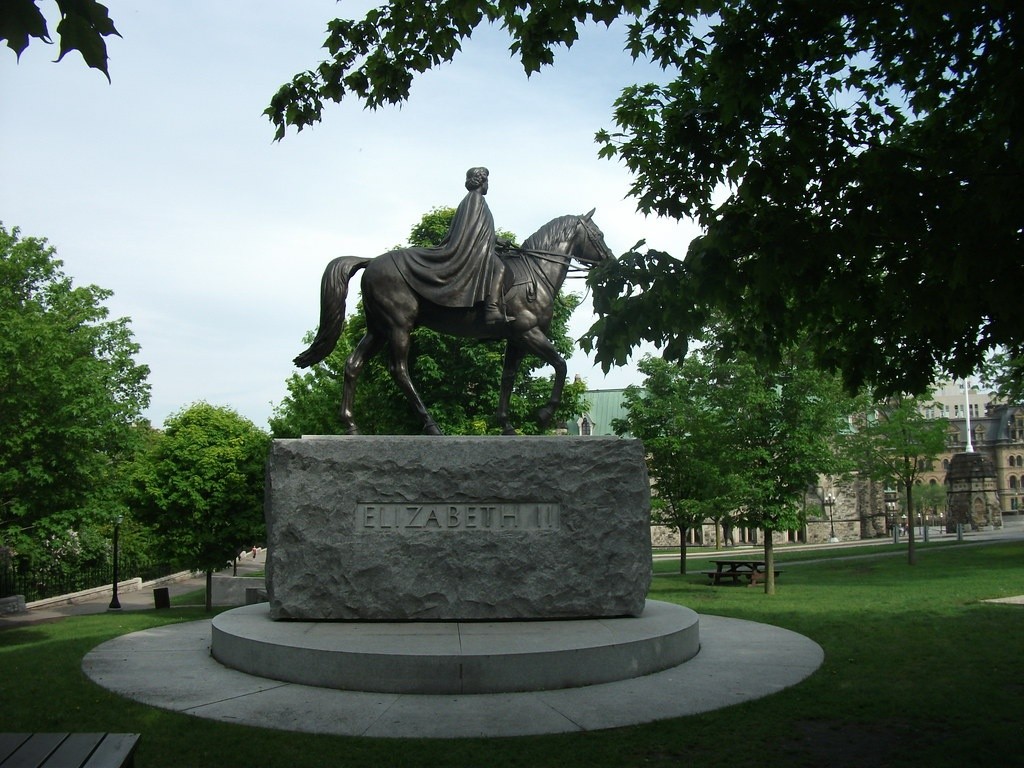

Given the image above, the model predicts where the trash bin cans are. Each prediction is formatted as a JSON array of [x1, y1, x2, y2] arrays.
[[153, 587, 170, 609]]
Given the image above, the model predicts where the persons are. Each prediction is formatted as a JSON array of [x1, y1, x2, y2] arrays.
[[252, 545, 256, 558], [390, 166, 516, 326]]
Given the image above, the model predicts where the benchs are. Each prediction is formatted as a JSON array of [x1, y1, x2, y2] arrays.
[[0, 732, 141, 768], [702, 570, 784, 585]]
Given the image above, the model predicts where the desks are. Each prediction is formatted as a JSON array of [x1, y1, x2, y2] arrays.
[[709, 559, 776, 586]]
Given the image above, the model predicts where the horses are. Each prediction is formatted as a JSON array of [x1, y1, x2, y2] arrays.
[[292, 208, 617, 437]]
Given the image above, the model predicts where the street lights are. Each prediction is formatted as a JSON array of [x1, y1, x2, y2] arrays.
[[107, 515, 125, 612], [825, 493, 839, 543]]
